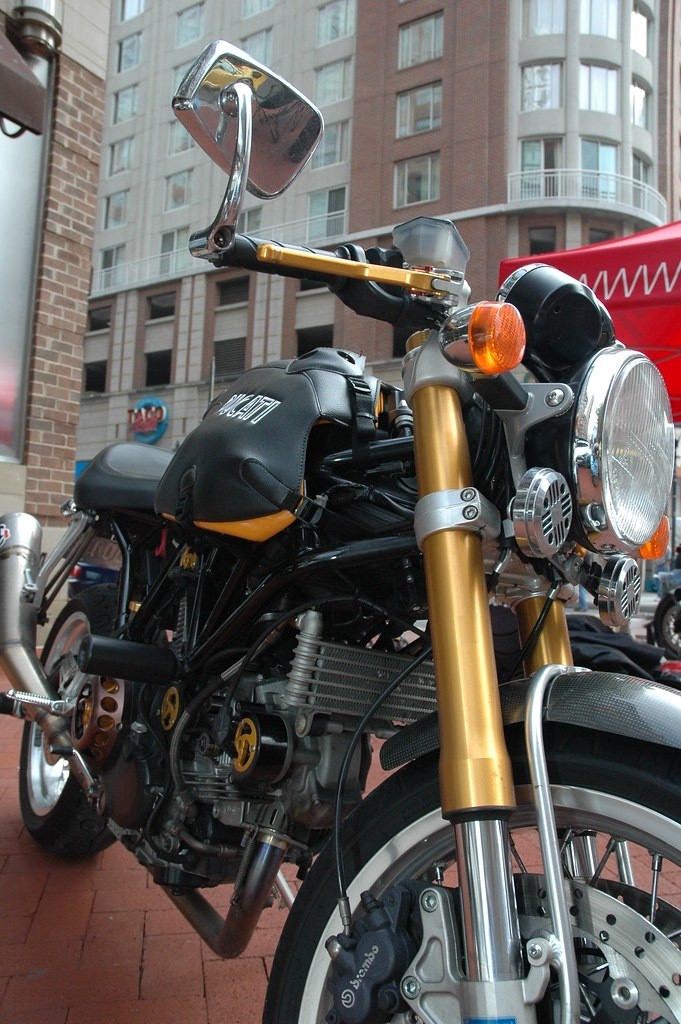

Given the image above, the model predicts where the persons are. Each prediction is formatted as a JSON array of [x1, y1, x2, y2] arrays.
[[574, 583, 589, 611], [654, 545, 671, 601]]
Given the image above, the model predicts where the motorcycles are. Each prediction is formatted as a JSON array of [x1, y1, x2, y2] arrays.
[[0, 42, 681, 1024]]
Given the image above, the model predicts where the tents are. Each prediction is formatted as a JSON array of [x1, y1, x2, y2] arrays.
[[497, 220, 681, 571]]
